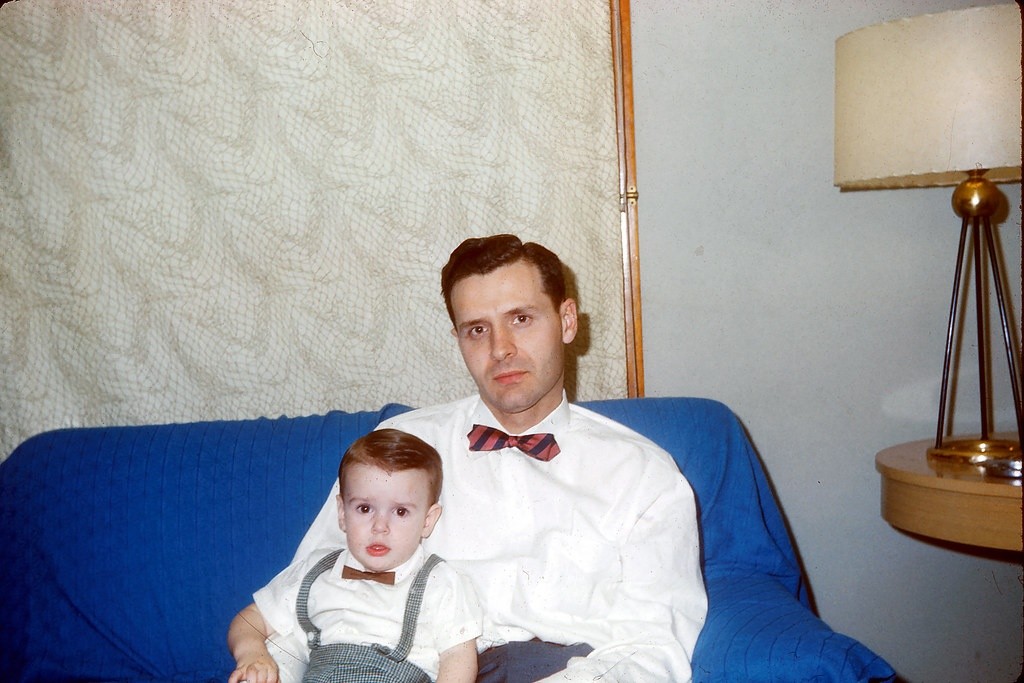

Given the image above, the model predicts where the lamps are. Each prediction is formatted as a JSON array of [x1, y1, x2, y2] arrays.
[[832, 0, 1024, 476]]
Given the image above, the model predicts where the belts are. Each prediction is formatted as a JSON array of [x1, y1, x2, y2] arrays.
[[477, 636, 568, 659]]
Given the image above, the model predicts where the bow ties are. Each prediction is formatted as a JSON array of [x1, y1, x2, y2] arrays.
[[467, 424, 561, 462], [341, 566, 395, 585]]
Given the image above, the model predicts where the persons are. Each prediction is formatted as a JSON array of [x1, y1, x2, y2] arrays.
[[228, 429, 481, 682], [242, 235, 708, 683]]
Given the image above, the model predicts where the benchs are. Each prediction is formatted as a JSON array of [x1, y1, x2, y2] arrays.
[[0, 398, 892, 682]]
